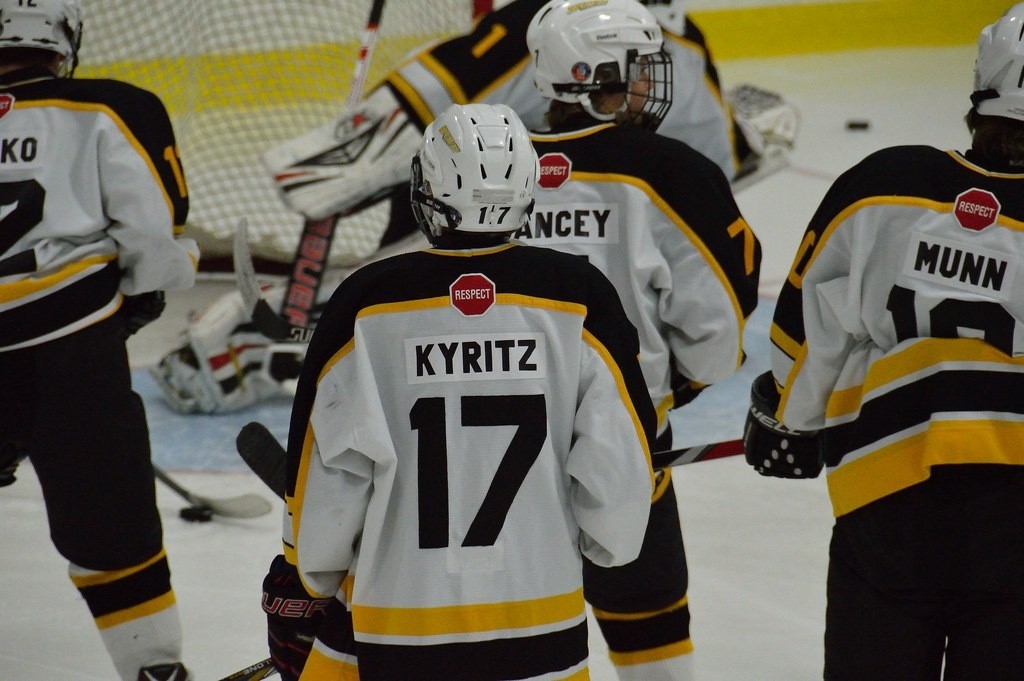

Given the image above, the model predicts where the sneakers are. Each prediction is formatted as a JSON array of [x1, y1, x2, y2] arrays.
[[149, 346, 216, 415]]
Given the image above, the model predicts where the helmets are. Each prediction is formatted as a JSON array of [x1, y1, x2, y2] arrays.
[[973, 1, 1024, 120], [526, 1, 672, 133], [0, 0, 82, 60], [410, 102, 542, 242]]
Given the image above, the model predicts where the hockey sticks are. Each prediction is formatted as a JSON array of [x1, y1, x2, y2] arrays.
[[219, 653, 279, 681], [230, 419, 748, 507], [151, 461, 277, 521], [228, 0, 390, 343]]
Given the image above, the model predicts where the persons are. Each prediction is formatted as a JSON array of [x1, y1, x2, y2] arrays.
[[742, 4, 1024, 681], [261, 104, 698, 680], [144, 0, 750, 415], [520, 1, 762, 681], [0, 0, 195, 681]]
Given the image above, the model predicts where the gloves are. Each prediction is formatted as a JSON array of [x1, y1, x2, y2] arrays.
[[260, 560, 347, 681], [742, 370, 823, 478]]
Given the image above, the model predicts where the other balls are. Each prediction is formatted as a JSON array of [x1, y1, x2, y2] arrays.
[[848, 122, 869, 129], [180, 507, 212, 522]]
